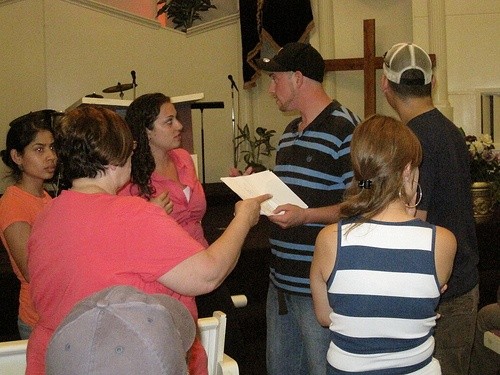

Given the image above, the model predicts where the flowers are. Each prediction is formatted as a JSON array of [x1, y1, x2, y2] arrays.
[[460, 128, 500, 184]]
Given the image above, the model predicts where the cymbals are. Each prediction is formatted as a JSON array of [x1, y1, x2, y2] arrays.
[[102, 83, 138, 93]]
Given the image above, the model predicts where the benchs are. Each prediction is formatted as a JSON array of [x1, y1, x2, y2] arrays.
[[0, 311, 240, 375]]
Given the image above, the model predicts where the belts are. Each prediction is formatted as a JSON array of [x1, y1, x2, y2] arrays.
[[277, 290, 289, 315]]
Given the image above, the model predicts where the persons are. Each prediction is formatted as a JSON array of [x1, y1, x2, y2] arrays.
[[24, 106, 273, 375], [229, 41, 362, 375], [0, 112, 57, 339], [382, 42, 480, 375], [117, 92, 247, 375], [310, 115, 457, 375]]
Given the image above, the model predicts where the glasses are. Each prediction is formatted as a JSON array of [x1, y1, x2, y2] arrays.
[[381, 51, 391, 68]]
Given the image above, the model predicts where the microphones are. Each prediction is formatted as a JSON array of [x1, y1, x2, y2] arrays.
[[131, 71, 137, 86], [227, 74, 238, 92]]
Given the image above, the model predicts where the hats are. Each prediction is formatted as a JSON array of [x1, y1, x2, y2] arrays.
[[47, 283, 195, 375], [257, 42, 325, 82], [383, 41, 435, 86]]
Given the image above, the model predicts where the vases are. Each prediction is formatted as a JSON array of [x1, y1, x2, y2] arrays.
[[471, 181, 496, 224]]
